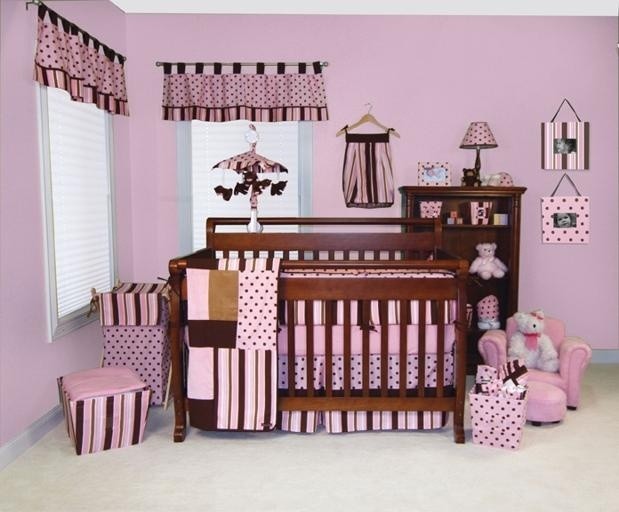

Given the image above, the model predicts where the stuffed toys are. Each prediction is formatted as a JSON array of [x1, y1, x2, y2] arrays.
[[506, 308, 560, 374], [467, 241, 509, 281], [460, 167, 482, 188]]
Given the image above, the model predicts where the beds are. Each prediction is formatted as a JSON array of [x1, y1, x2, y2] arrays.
[[168, 218, 468, 444]]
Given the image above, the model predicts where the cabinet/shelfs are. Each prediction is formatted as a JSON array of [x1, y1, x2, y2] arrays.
[[398, 186, 528, 375]]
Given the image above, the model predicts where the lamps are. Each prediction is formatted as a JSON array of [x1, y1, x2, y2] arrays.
[[460, 122, 498, 186]]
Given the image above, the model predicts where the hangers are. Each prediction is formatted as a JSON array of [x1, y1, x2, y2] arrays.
[[334, 102, 402, 140]]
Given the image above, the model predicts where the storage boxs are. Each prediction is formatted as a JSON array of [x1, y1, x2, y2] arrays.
[[468, 382, 528, 451], [92, 280, 172, 412]]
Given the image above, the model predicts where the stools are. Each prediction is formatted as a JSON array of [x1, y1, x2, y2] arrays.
[[56, 366, 152, 456], [518, 379, 567, 426]]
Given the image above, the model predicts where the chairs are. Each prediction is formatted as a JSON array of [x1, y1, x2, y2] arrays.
[[477, 314, 593, 410]]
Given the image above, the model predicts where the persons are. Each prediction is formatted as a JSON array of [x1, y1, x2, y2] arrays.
[[553, 138, 578, 154], [553, 213, 577, 228], [424, 168, 446, 182]]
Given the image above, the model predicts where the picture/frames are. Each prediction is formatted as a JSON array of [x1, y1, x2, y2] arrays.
[[417, 162, 452, 187], [539, 196, 592, 244], [540, 121, 590, 171]]
